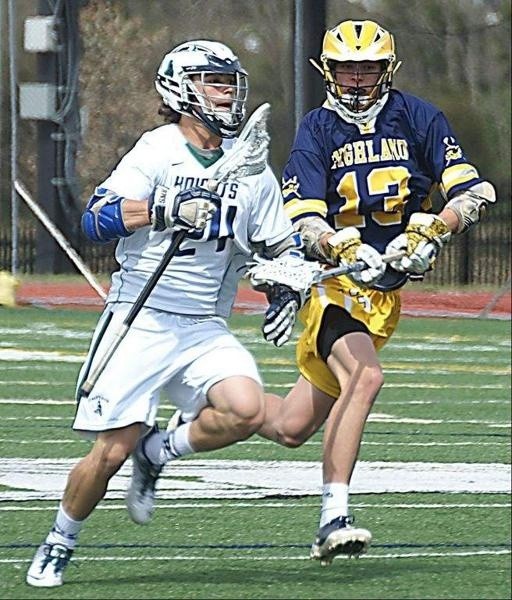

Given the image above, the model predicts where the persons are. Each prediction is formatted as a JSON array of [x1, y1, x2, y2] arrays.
[[164, 18, 499, 564], [23, 35, 303, 592]]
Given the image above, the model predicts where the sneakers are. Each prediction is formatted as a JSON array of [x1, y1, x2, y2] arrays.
[[24, 527, 78, 588], [310, 514, 372, 561], [126, 423, 166, 524], [166, 409, 186, 433]]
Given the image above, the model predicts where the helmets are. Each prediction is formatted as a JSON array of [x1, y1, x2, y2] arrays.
[[320, 18, 397, 123], [153, 39, 248, 139]]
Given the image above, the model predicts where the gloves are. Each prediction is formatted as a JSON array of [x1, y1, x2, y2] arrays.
[[385, 212, 452, 275], [148, 184, 221, 244], [261, 284, 301, 348], [326, 226, 384, 284]]
